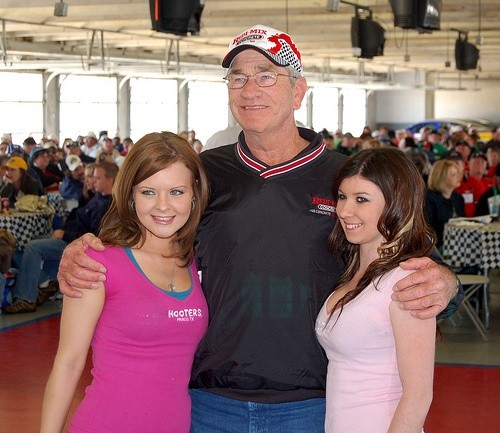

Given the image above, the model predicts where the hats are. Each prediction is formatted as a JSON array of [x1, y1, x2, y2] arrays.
[[5, 144, 25, 158], [100, 131, 107, 136], [66, 155, 83, 171], [342, 132, 354, 138], [441, 151, 463, 160], [44, 142, 57, 150], [65, 141, 79, 147], [221, 25, 304, 75], [101, 135, 112, 142], [86, 132, 96, 139], [29, 146, 50, 160], [2, 157, 27, 171]]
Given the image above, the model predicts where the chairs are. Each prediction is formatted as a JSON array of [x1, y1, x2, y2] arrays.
[[436, 274, 493, 343]]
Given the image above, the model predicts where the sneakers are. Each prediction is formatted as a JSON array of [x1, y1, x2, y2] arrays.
[[37, 278, 59, 306], [2, 299, 36, 314]]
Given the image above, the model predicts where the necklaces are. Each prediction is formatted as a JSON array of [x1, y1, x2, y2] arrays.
[[143, 245, 177, 295]]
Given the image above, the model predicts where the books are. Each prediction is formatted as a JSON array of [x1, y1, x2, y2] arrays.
[[448, 215, 492, 227]]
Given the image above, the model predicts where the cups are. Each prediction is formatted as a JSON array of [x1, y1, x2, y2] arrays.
[[488, 194, 500, 217]]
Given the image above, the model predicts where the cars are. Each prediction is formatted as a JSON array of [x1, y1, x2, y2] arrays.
[[404, 117, 498, 143]]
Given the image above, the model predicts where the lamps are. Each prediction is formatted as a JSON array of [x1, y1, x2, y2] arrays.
[[388, 0, 442, 35], [339, 0, 387, 60], [449, 27, 480, 71], [149, 0, 206, 38]]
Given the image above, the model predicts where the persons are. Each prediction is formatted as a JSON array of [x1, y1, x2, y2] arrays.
[[37, 131, 210, 433], [312, 145, 437, 433], [0, 131, 137, 314], [315, 122, 500, 330], [57, 24, 464, 433], [180, 130, 203, 160]]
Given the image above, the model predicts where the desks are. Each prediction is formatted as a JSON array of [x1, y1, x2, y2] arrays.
[[443, 213, 500, 330], [0, 207, 57, 250], [44, 183, 66, 223]]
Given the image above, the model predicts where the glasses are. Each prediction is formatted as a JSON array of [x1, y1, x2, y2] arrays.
[[223, 70, 297, 89]]
